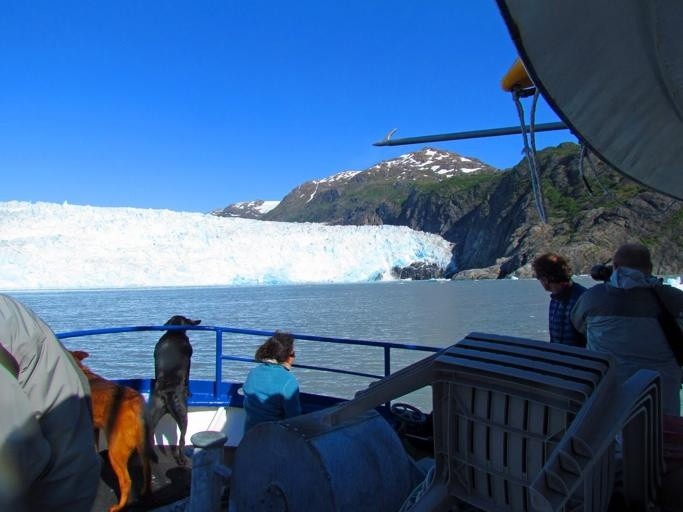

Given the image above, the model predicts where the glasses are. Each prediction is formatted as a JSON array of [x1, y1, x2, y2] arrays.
[[290, 352, 295, 357]]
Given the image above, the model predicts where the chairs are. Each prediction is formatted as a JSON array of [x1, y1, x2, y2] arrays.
[[324, 330, 670, 512]]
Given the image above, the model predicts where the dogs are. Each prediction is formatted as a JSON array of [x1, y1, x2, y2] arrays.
[[147, 315, 201, 466], [66, 349, 153, 512]]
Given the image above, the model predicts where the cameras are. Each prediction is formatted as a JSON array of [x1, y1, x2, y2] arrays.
[[591, 262, 612, 283]]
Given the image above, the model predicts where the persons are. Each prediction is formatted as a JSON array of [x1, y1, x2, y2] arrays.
[[530, 250, 591, 349], [0, 288, 102, 512], [241, 330, 302, 440], [568, 242, 681, 418]]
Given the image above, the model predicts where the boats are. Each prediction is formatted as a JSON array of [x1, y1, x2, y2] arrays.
[[36, 1, 682, 512]]
